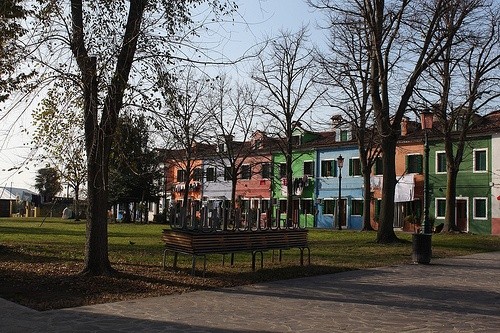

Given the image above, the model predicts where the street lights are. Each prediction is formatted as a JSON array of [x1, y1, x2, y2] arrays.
[[337, 154, 345, 231], [419, 101, 435, 235]]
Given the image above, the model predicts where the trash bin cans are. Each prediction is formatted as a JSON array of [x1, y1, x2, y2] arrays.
[[411, 233, 432, 266]]
